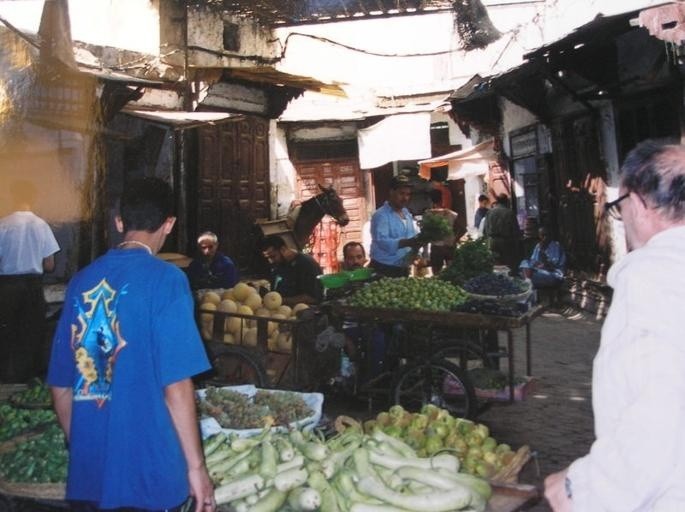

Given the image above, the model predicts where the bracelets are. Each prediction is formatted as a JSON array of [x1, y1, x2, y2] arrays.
[[564, 477, 573, 498]]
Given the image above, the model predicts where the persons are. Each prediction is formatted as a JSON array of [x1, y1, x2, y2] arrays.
[[366, 174, 429, 280], [514, 225, 567, 290], [44, 174, 219, 511], [482, 193, 524, 266], [539, 129, 685, 510], [326, 242, 370, 301], [259, 235, 325, 307], [421, 188, 461, 275], [521, 217, 538, 258], [0, 179, 62, 382], [186, 231, 239, 291], [474, 194, 491, 228]]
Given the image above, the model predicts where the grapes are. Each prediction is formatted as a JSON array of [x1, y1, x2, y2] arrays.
[[195, 388, 312, 428]]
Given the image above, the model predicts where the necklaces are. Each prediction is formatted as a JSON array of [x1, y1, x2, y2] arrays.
[[115, 241, 152, 256]]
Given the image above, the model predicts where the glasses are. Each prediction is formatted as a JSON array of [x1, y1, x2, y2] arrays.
[[603, 187, 648, 224]]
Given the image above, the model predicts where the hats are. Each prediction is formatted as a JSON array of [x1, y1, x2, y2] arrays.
[[389, 174, 417, 190]]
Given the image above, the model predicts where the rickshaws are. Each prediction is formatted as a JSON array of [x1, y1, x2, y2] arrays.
[[192, 267, 545, 423]]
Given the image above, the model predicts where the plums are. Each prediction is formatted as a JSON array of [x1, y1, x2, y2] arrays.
[[462, 271, 524, 294]]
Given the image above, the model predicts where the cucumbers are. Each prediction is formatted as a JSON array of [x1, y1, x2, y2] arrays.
[[201, 423, 491, 511]]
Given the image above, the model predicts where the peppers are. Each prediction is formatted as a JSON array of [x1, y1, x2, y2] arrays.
[[0, 374, 70, 483]]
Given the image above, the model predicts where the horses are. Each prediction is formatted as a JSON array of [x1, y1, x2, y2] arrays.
[[286, 182, 350, 250]]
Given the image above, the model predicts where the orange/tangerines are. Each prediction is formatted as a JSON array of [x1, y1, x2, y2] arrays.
[[344, 274, 463, 311]]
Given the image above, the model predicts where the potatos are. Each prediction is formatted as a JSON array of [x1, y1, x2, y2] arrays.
[[194, 282, 311, 355]]
